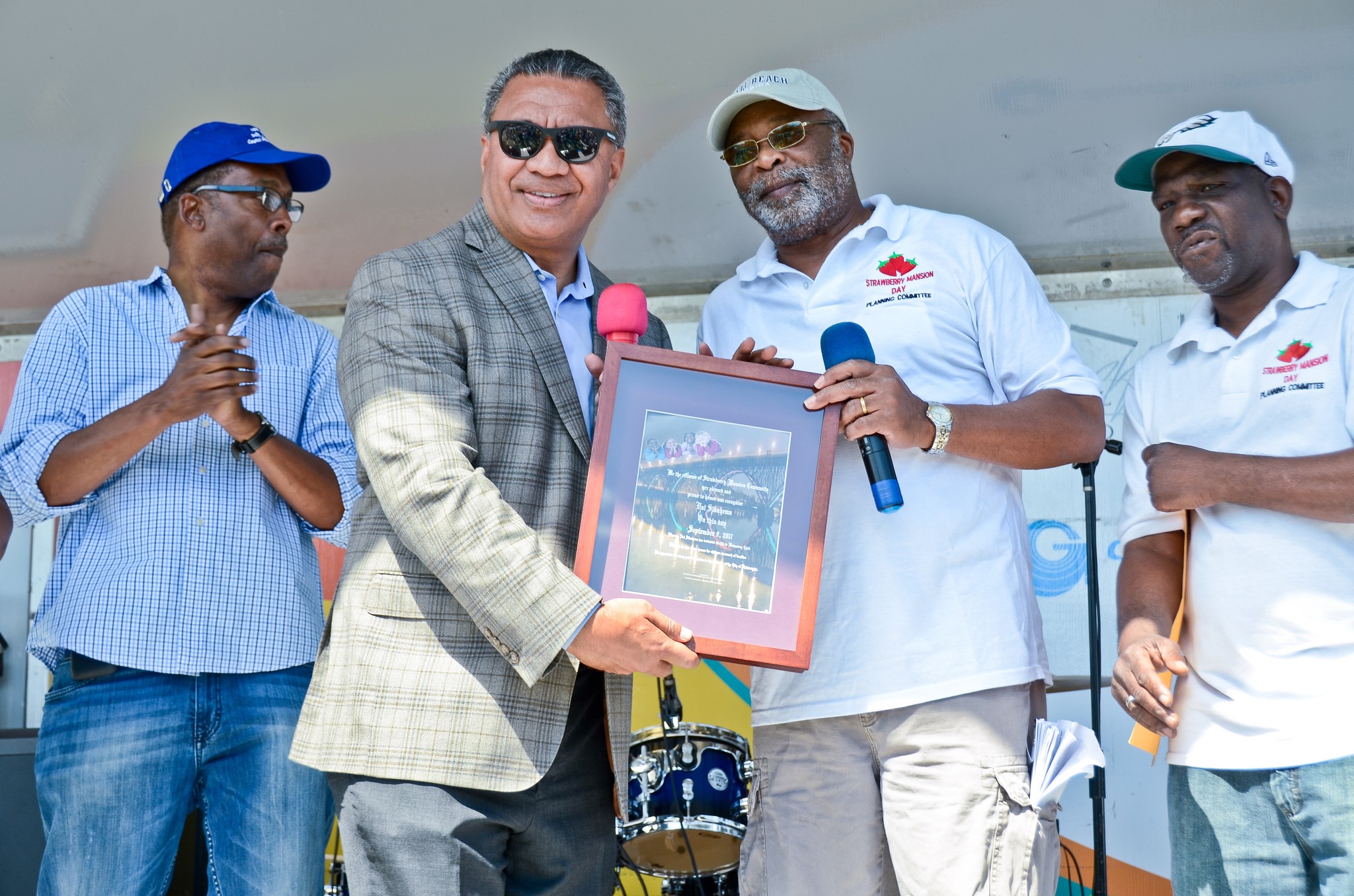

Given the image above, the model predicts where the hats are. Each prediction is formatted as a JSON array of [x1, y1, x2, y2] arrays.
[[707, 68, 850, 151], [1115, 110, 1294, 193], [158, 121, 331, 212]]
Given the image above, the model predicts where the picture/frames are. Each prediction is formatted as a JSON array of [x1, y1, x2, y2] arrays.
[[574, 340, 840, 675]]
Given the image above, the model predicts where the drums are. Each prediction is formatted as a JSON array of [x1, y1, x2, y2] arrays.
[[662, 868, 739, 896], [326, 860, 349, 896], [615, 722, 755, 878]]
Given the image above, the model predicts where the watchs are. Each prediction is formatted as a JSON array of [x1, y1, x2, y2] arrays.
[[230, 411, 277, 461], [919, 401, 953, 455]]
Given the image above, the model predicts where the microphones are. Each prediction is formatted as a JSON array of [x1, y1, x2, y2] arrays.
[[820, 320, 904, 515], [664, 669, 683, 724], [596, 283, 648, 346]]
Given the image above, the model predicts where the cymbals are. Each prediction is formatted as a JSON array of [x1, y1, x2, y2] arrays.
[[1046, 673, 1113, 694]]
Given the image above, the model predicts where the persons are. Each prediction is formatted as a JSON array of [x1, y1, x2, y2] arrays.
[[0, 121, 362, 896], [585, 68, 1105, 896], [288, 49, 795, 896], [1112, 110, 1354, 896]]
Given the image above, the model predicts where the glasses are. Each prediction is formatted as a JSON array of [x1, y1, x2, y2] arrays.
[[191, 185, 305, 222], [484, 120, 622, 164], [720, 121, 837, 167]]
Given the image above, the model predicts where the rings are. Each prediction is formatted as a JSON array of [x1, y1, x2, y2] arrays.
[[1126, 695, 1136, 707], [860, 397, 868, 416]]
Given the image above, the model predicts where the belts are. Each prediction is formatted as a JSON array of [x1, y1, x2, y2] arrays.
[[47, 660, 116, 689]]
